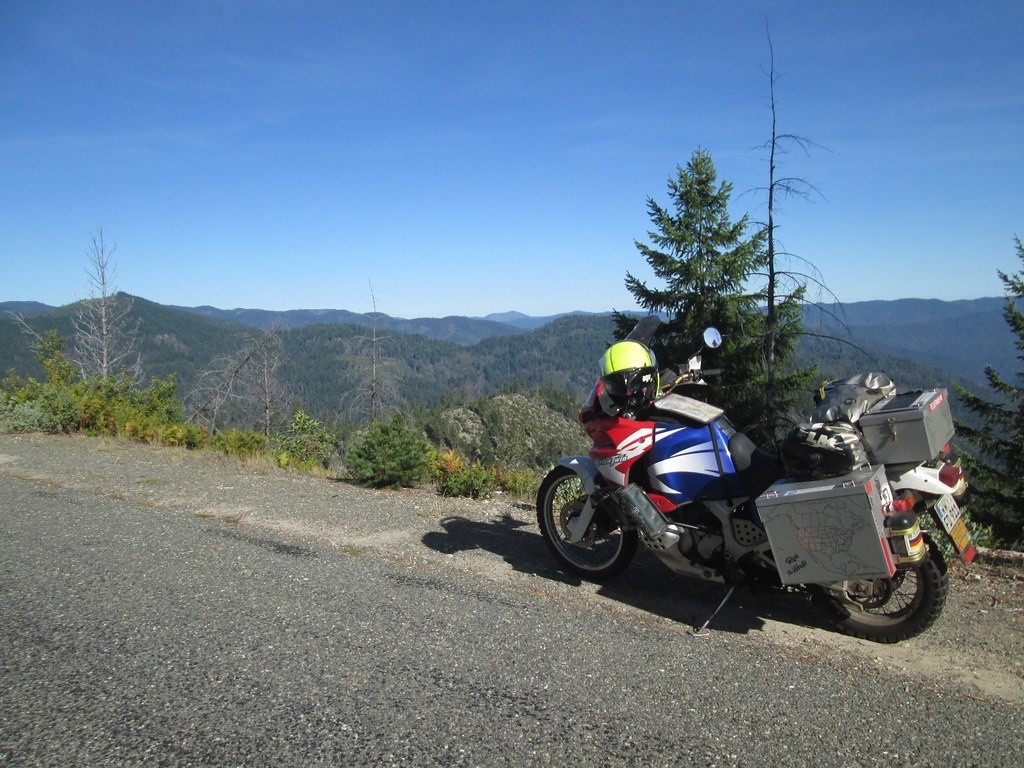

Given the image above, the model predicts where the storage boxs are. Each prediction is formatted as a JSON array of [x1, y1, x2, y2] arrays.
[[755, 463, 898, 587], [857, 388, 954, 466]]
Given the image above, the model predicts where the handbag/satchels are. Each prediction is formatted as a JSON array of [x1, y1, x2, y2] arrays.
[[779, 369, 896, 480]]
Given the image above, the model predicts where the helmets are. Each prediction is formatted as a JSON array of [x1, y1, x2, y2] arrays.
[[599, 340, 659, 401]]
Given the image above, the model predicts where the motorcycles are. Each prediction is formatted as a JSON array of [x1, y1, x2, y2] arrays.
[[536, 315, 978, 645]]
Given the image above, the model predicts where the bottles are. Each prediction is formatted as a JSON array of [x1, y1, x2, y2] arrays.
[[886, 499, 928, 571], [615, 482, 669, 541], [941, 445, 967, 501]]
